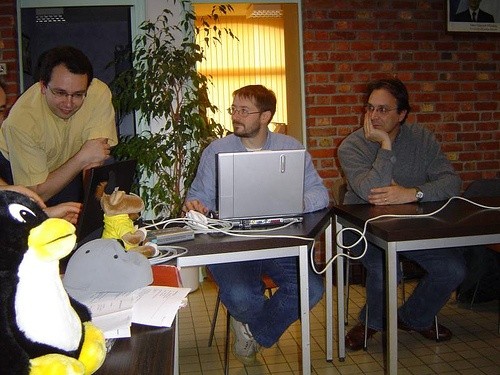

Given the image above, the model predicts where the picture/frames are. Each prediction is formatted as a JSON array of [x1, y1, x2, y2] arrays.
[[444, 0, 500, 36]]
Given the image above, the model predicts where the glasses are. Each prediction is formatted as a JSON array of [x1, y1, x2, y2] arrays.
[[365, 105, 398, 114], [47, 84, 85, 98], [228, 107, 261, 117]]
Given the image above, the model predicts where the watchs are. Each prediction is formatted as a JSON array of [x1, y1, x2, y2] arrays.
[[415, 188, 424, 201]]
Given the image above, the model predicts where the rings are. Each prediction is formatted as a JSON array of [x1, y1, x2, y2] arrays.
[[385, 197, 387, 202]]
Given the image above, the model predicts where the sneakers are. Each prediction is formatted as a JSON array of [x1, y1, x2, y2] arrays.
[[228, 315, 260, 366]]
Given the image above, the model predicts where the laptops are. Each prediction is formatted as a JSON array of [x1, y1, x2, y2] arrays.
[[76, 157, 137, 242], [211, 148, 307, 227]]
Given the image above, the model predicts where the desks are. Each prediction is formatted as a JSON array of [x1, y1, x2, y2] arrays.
[[333, 196, 500, 375], [161, 207, 334, 375], [58, 265, 180, 375]]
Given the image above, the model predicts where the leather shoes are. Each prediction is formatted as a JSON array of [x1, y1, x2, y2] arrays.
[[397, 309, 452, 341], [346, 319, 383, 351]]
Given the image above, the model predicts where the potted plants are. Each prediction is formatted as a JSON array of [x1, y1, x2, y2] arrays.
[[103, 0, 240, 289]]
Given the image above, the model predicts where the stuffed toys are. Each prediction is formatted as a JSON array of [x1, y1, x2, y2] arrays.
[[0, 190, 106, 375], [64, 238, 154, 291], [97, 172, 159, 258]]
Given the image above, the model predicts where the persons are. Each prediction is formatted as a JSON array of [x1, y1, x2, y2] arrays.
[[179, 85, 329, 365], [0, 47, 118, 208], [338, 78, 461, 351], [0, 83, 83, 226]]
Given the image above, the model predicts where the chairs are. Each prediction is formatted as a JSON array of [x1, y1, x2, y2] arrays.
[[206, 180, 409, 375]]
